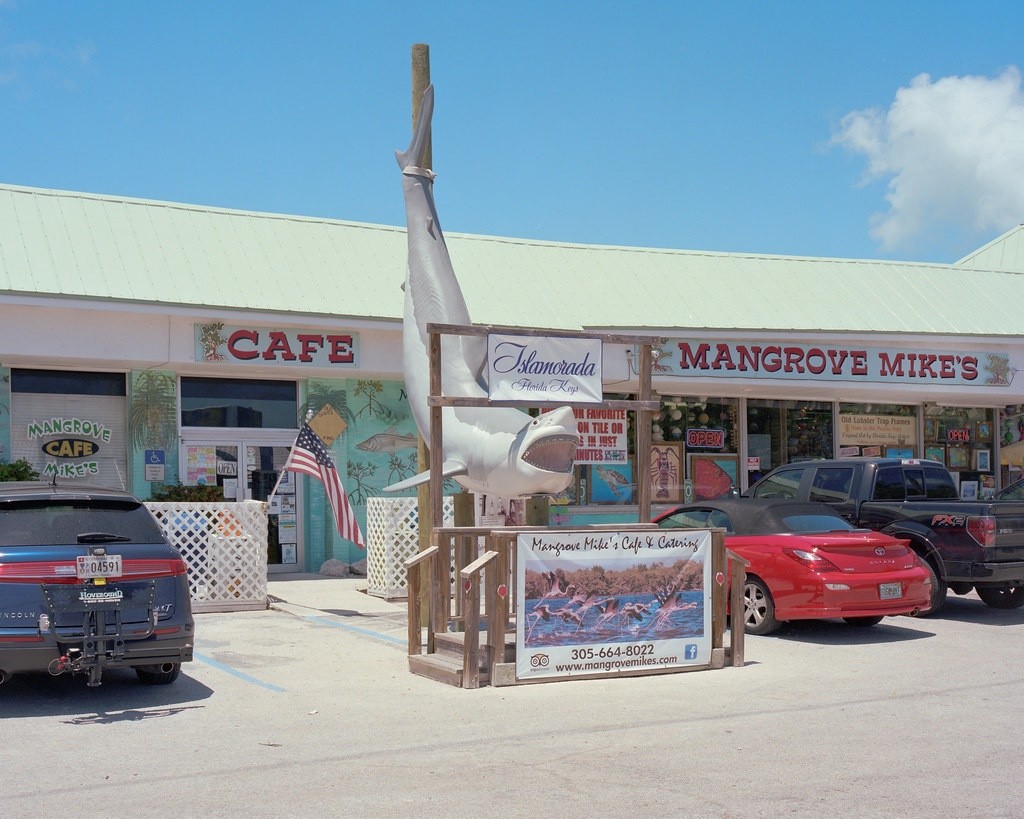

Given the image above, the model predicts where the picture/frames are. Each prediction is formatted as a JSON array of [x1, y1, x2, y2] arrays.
[[651, 441, 685, 504], [691, 455, 738, 504], [882, 420, 993, 473], [586, 454, 637, 505]]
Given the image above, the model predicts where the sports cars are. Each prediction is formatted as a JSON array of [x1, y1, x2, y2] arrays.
[[648, 496, 934, 636]]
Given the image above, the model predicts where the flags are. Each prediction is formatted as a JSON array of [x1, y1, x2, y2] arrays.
[[283, 422, 366, 549]]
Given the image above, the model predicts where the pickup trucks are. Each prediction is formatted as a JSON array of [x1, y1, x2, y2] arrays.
[[737, 456, 1024, 618]]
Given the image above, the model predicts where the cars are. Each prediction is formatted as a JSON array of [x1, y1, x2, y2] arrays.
[[0, 480, 195, 686]]
